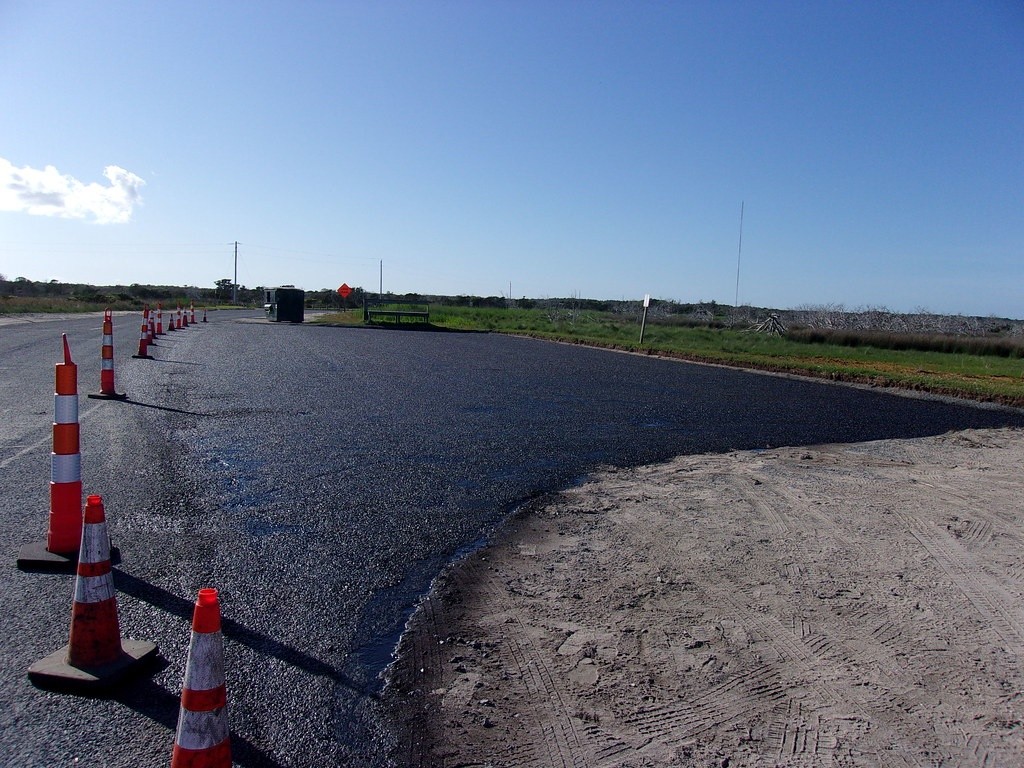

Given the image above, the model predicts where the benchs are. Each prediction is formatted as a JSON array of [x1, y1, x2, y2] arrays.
[[361, 297, 431, 326]]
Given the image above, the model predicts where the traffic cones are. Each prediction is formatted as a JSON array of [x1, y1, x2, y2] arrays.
[[182, 306, 189, 327], [146, 317, 159, 345], [167, 314, 176, 331], [170, 588, 233, 768], [29, 494, 159, 690], [201, 309, 209, 322]]
